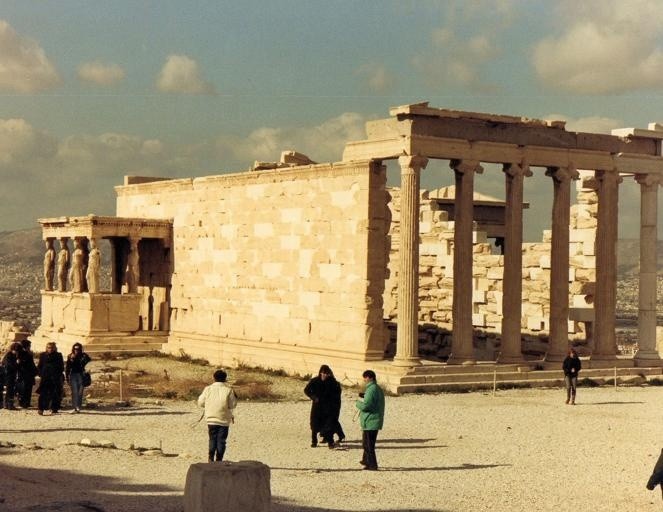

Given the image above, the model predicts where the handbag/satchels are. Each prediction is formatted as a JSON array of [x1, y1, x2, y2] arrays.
[[81, 373, 91, 387]]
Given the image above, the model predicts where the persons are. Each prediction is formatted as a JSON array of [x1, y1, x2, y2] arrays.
[[354, 370, 385, 470], [302, 364, 335, 448], [562, 347, 581, 405], [42, 238, 140, 295], [320, 368, 345, 444], [0, 338, 91, 416], [645, 447, 662, 499], [196, 369, 237, 463]]
